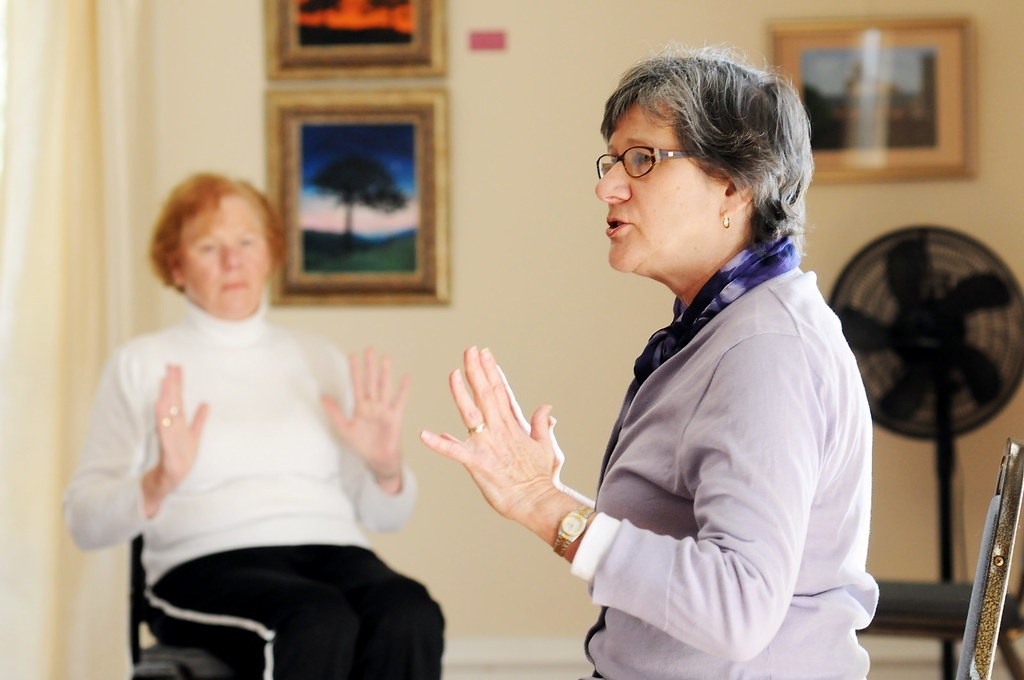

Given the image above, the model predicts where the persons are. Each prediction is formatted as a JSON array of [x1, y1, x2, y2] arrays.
[[418, 47, 881, 680], [65, 172, 446, 680]]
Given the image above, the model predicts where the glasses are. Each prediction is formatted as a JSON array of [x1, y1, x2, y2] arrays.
[[596, 146, 710, 179]]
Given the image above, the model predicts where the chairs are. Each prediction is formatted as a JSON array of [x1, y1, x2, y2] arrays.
[[129, 535, 277, 680], [952, 437, 1023, 680]]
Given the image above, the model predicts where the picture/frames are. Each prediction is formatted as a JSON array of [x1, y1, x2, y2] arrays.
[[267, 86, 453, 309], [767, 14, 980, 182], [264, 1, 448, 81]]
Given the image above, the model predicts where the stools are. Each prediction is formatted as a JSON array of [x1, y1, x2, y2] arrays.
[[858, 573, 1024, 680]]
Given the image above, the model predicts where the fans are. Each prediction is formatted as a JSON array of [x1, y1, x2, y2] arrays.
[[826, 224, 1024, 680]]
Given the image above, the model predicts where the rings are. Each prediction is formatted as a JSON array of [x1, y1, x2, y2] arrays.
[[170, 406, 180, 416], [160, 418, 171, 428], [469, 422, 486, 435]]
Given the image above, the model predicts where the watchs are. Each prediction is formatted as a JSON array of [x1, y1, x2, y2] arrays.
[[553, 500, 595, 557]]
